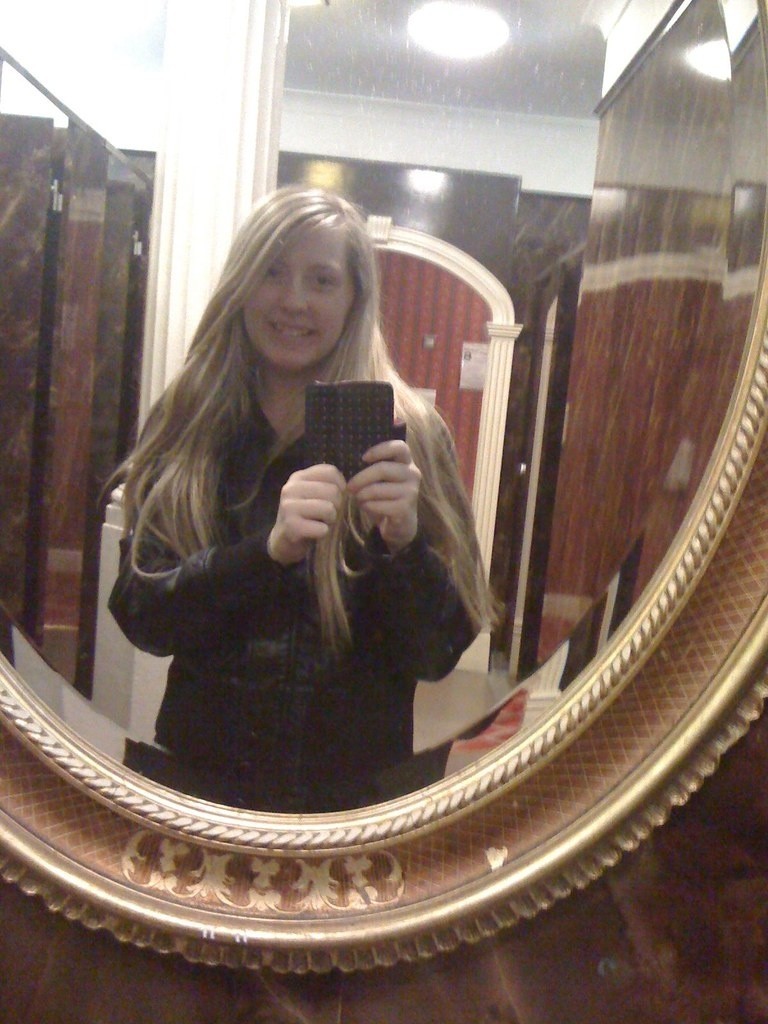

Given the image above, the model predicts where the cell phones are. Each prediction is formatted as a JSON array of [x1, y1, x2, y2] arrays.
[[304, 378, 396, 481]]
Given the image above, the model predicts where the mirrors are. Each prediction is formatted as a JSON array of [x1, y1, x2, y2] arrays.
[[1, 0, 768, 978]]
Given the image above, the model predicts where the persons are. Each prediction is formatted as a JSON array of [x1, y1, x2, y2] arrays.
[[95, 183, 505, 786]]
